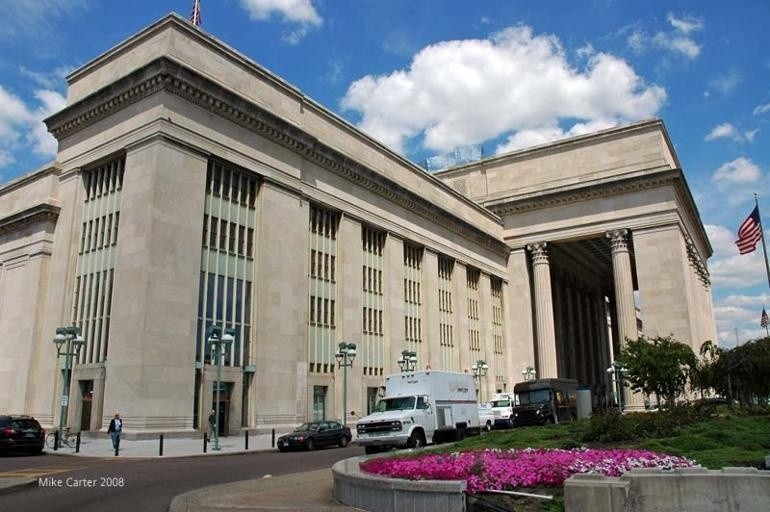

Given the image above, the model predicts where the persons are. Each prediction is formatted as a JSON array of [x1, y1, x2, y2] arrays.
[[208, 410, 218, 442], [106, 414, 122, 452]]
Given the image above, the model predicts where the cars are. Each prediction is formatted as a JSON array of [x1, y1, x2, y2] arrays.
[[277, 421, 352, 451]]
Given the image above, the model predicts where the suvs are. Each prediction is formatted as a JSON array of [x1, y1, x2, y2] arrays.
[[0, 415, 45, 455]]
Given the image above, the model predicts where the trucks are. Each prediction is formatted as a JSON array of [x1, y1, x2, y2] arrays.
[[351, 371, 479, 454], [490, 393, 520, 428]]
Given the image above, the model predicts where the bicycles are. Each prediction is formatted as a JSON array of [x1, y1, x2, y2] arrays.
[[46, 427, 81, 448]]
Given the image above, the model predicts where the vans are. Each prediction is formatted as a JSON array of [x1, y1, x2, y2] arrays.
[[513, 378, 579, 428], [478, 403, 495, 431]]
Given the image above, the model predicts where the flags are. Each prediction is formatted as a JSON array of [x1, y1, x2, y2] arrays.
[[734, 207, 761, 256], [190, 0, 202, 25], [759, 308, 770, 328]]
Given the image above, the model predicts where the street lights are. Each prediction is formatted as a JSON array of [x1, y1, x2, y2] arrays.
[[335, 342, 356, 426], [522, 366, 536, 382], [612, 361, 626, 413], [53, 326, 84, 448], [472, 360, 488, 406], [207, 326, 235, 450], [398, 350, 417, 372]]
